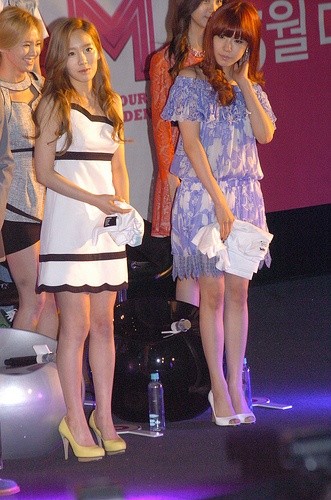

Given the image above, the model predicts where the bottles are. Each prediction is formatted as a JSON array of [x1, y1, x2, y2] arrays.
[[241, 357, 253, 409], [148, 373, 165, 433]]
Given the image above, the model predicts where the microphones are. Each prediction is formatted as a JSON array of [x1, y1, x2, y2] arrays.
[[4, 353, 56, 366], [142, 318, 192, 338]]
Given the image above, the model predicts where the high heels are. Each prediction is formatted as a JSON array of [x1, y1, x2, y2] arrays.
[[89, 410, 126, 456], [207, 390, 256, 426], [59, 415, 105, 462]]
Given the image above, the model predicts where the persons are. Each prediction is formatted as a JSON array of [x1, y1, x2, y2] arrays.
[[33, 18, 131, 458], [161, 0, 276, 425], [0, 7, 59, 337], [150, 0, 222, 238]]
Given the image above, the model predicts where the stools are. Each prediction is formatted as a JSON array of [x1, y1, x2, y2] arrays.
[[0, 329, 86, 462], [89, 297, 211, 424], [118, 219, 177, 304]]
